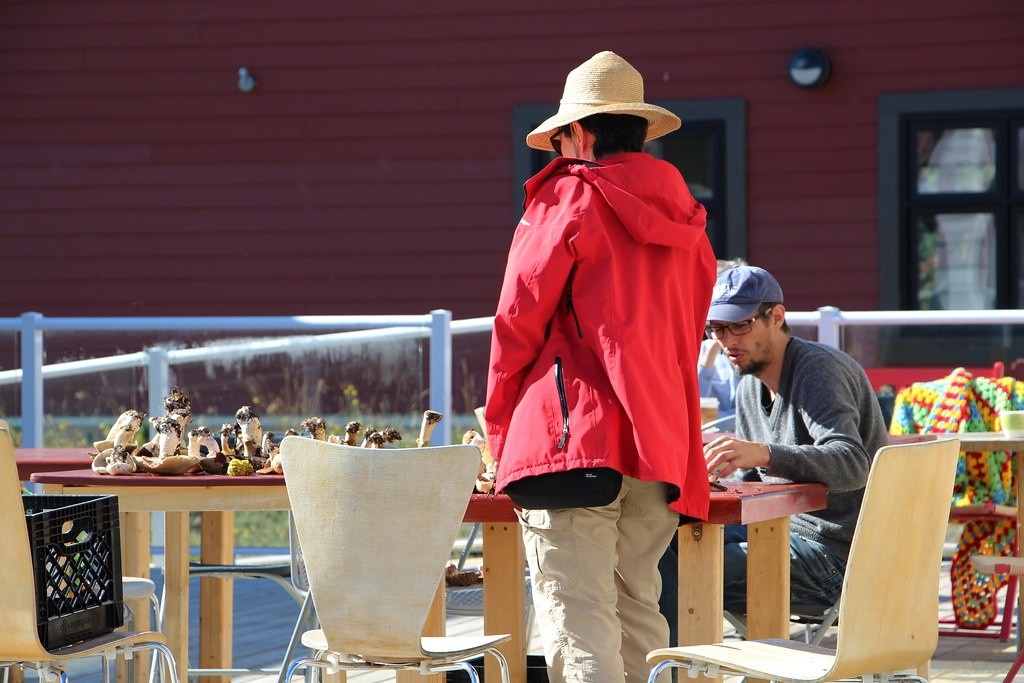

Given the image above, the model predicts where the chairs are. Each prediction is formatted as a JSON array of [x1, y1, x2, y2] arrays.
[[0, 420, 180, 683], [447, 523, 536, 653], [279, 435, 513, 683], [647, 437, 961, 683], [865, 362, 1023, 642]]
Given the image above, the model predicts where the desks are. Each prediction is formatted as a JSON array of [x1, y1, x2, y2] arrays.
[[703, 432, 936, 446], [13, 446, 828, 683], [936, 432, 1024, 651]]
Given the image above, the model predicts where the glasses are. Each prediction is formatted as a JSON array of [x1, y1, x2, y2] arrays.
[[550, 127, 566, 156], [704, 305, 775, 340]]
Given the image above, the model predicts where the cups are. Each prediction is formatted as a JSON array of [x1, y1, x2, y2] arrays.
[[700, 397, 720, 427], [999, 411, 1024, 437]]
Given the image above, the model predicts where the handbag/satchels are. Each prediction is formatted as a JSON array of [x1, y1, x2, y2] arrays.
[[507, 466, 623, 508]]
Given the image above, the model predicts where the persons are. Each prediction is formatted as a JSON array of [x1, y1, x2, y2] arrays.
[[697, 326, 742, 430], [657, 265, 890, 648], [484, 51, 717, 683]]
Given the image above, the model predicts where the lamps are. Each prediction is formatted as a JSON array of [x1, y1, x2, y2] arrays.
[[788, 47, 831, 88]]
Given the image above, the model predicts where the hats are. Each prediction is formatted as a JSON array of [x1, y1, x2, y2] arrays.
[[525, 51, 682, 152], [704, 264, 783, 322]]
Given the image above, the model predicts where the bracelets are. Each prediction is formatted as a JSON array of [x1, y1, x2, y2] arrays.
[[759, 444, 771, 474]]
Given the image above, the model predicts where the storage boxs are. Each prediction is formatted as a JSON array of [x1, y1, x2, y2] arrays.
[[21, 495, 124, 648]]
